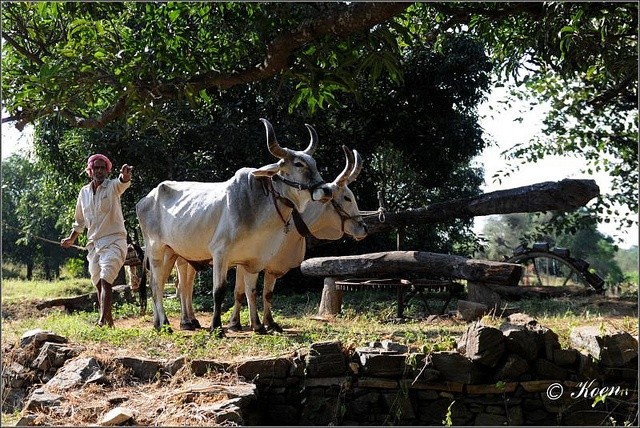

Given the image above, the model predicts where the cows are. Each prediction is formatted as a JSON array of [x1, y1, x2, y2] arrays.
[[174, 144, 370, 335], [134, 117, 334, 336]]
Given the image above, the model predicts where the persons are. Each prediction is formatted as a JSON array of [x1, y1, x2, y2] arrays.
[[61, 153, 134, 330]]
